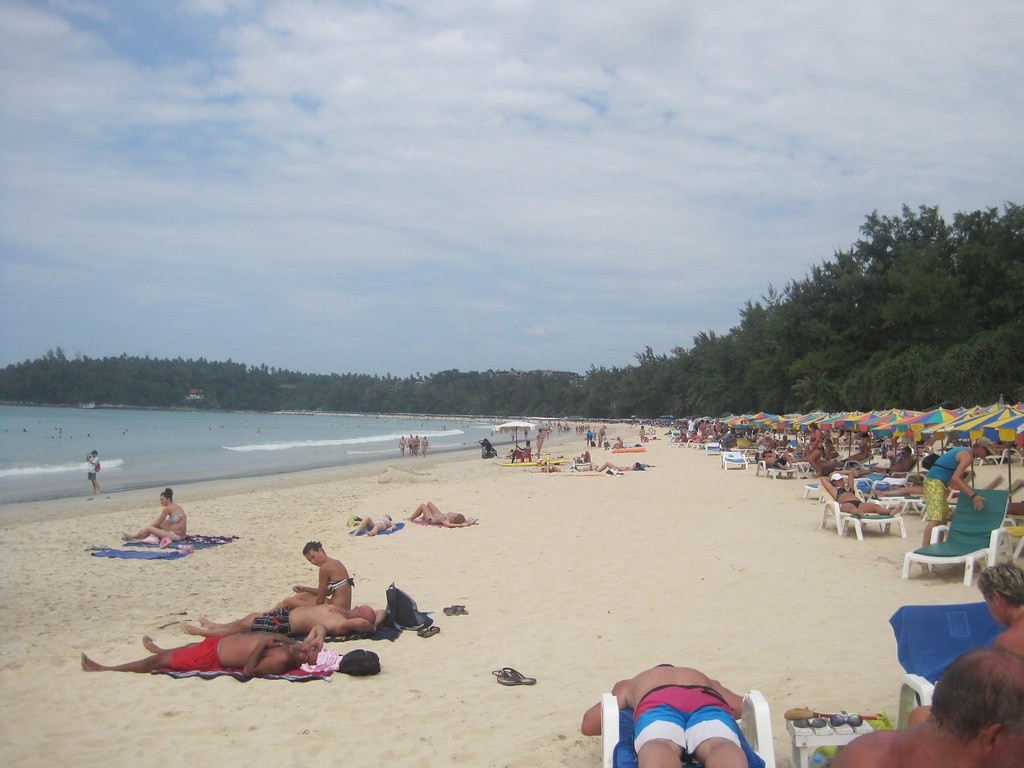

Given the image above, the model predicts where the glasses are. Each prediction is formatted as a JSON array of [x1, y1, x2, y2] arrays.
[[793, 717, 826, 728], [830, 714, 862, 727]]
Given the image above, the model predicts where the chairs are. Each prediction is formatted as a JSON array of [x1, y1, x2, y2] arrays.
[[570, 431, 1024, 768], [521, 449, 532, 463]]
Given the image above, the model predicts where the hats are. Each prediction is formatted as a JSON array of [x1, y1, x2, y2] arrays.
[[90, 450, 98, 454], [830, 473, 844, 481], [977, 437, 995, 455]]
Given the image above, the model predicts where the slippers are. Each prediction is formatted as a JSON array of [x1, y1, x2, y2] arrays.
[[416, 625, 440, 638], [492, 667, 536, 685]]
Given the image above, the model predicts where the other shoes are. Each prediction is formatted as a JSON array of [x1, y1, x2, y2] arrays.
[[443, 605, 468, 616]]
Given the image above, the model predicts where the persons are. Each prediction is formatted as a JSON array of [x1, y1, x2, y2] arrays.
[[922, 437, 995, 548], [123, 487, 187, 541], [407, 501, 476, 527], [352, 515, 396, 536], [829, 649, 1024, 768], [907, 562, 1024, 728], [506, 441, 531, 463], [536, 428, 544, 462], [86, 450, 101, 494], [807, 423, 824, 477], [820, 470, 901, 517], [273, 541, 355, 611], [581, 664, 749, 768], [81, 624, 327, 677], [0, 412, 1024, 768]]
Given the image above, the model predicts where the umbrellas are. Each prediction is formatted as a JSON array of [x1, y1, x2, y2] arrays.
[[493, 420, 537, 463], [724, 402, 1024, 450]]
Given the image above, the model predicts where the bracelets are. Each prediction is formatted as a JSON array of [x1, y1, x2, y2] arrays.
[[970, 493, 977, 499]]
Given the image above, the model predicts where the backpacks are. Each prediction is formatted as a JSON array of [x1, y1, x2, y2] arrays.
[[383, 585, 434, 631], [95, 463, 100, 472]]
